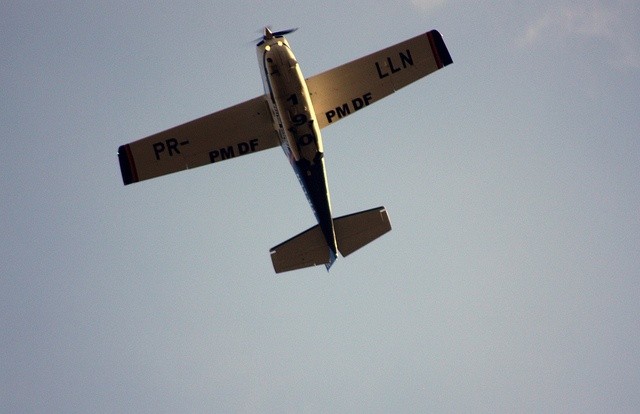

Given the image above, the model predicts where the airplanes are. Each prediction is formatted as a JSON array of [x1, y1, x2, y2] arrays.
[[118, 28, 453, 273]]
